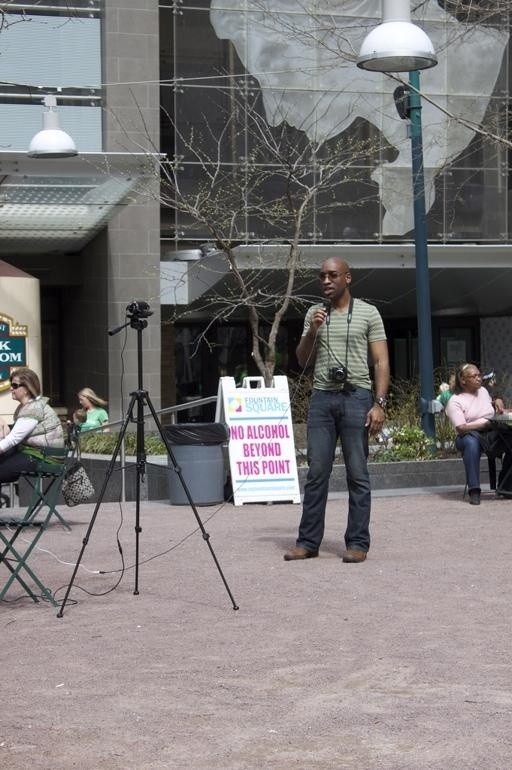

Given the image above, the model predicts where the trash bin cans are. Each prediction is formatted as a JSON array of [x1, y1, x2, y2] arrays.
[[161, 423, 230, 506]]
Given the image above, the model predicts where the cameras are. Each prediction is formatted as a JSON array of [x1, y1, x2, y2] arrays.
[[328, 367, 347, 380], [108, 301, 153, 336]]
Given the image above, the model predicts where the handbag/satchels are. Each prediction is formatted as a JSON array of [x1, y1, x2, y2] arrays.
[[58, 423, 97, 510]]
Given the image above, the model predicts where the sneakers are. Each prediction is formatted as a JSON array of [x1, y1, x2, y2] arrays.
[[283, 543, 319, 562], [494, 487, 512, 499], [469, 488, 481, 506], [341, 547, 369, 565]]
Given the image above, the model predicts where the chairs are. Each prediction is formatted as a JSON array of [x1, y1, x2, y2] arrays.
[[458, 448, 497, 499], [16, 424, 83, 519], [1, 447, 69, 606]]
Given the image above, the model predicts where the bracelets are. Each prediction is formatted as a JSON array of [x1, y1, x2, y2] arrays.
[[374, 393, 389, 413]]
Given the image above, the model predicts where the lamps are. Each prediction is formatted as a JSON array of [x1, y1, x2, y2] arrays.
[[27, 93, 79, 158], [356, 0, 440, 73]]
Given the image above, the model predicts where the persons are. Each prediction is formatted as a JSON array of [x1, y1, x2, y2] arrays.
[[72, 384, 111, 433], [282, 256, 390, 564], [445, 356, 512, 506], [1, 366, 66, 483], [436, 366, 497, 409]]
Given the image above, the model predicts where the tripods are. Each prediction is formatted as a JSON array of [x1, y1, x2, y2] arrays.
[[57, 331, 239, 619]]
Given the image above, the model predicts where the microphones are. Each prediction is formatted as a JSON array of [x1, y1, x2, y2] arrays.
[[323, 294, 336, 313]]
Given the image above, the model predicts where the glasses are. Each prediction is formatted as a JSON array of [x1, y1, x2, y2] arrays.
[[9, 382, 26, 390]]
